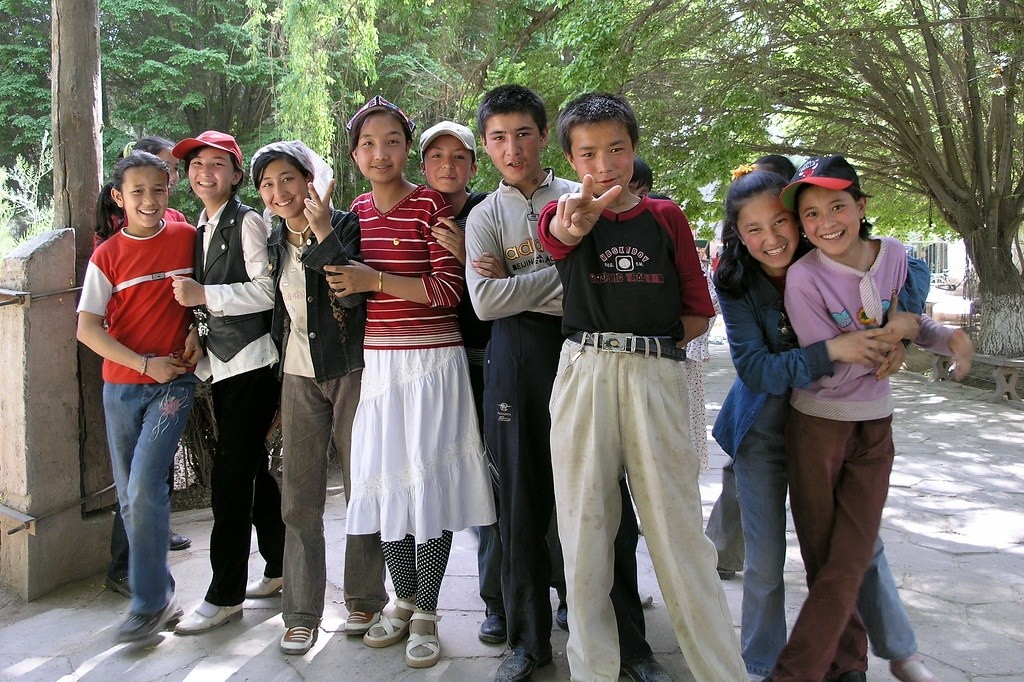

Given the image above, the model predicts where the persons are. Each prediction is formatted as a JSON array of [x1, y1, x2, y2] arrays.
[[763, 155, 974, 682], [323, 94, 498, 669], [467, 86, 682, 682], [248, 142, 391, 657], [418, 120, 571, 644], [75, 137, 199, 643], [169, 129, 287, 636], [536, 91, 755, 681], [706, 153, 930, 682]]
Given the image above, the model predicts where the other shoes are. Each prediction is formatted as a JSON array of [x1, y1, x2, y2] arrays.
[[106, 568, 131, 597], [118, 594, 183, 642], [637, 590, 652, 607], [169, 529, 192, 549], [716, 566, 736, 580], [889, 657, 940, 682]]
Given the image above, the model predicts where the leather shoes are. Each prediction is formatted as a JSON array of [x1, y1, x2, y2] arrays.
[[556, 596, 569, 628], [244, 574, 283, 598], [495, 644, 553, 682], [621, 653, 675, 682], [175, 604, 244, 633], [479, 614, 507, 643]]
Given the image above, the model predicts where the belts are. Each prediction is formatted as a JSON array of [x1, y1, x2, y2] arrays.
[[569, 330, 686, 362]]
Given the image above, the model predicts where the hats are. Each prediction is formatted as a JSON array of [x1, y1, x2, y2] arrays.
[[778, 155, 860, 211], [172, 130, 242, 167], [345, 95, 415, 142], [419, 121, 476, 164]]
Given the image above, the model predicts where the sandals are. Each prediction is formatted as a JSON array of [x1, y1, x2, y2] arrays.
[[363, 598, 418, 648], [405, 612, 442, 667]]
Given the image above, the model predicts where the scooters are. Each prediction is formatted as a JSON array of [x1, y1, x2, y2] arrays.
[[928, 262, 957, 291]]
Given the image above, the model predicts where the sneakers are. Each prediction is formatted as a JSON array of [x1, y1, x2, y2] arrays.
[[345, 606, 382, 635], [280, 619, 320, 654]]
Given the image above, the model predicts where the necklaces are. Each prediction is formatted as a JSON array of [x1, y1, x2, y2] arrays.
[[284, 218, 310, 246]]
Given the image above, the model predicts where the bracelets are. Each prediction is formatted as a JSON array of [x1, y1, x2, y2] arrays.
[[378, 270, 383, 294], [141, 354, 148, 376]]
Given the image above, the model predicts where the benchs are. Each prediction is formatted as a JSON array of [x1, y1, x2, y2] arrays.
[[928, 350, 1024, 404]]
